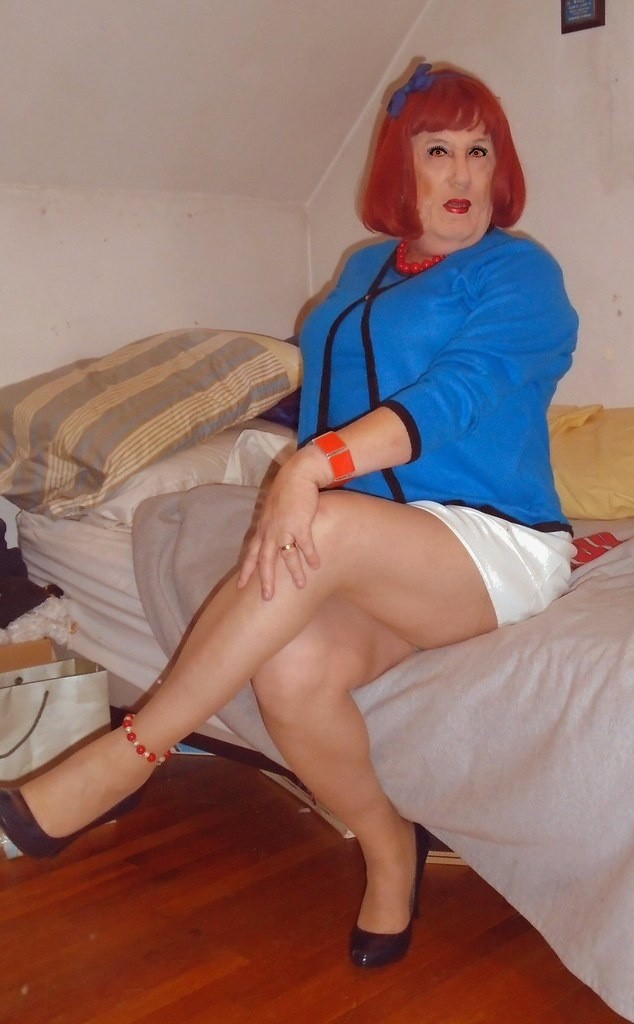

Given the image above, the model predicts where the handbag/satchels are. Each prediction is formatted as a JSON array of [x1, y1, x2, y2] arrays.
[[0, 657, 113, 787]]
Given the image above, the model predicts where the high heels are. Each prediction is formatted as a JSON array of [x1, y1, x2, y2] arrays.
[[347, 821, 431, 970], [0, 774, 156, 858]]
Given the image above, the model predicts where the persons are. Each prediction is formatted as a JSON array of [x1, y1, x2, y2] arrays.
[[1, 63, 579, 967]]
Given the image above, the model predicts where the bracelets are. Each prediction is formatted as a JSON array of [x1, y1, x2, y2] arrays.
[[305, 430, 355, 488]]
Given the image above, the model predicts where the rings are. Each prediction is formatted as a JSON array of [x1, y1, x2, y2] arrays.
[[280, 543, 296, 552]]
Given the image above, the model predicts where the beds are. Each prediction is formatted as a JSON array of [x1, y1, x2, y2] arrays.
[[15, 404, 634, 814]]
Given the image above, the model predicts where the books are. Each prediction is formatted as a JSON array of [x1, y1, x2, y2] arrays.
[[172, 742, 215, 756], [260, 769, 469, 865]]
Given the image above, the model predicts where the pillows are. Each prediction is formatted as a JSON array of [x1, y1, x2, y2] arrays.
[[0, 328, 304, 519], [87, 418, 298, 521]]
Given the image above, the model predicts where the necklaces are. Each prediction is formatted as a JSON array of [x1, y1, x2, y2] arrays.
[[396, 238, 446, 273]]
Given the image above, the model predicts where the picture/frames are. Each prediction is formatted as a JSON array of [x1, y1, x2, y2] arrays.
[[561, 0, 605, 34]]
